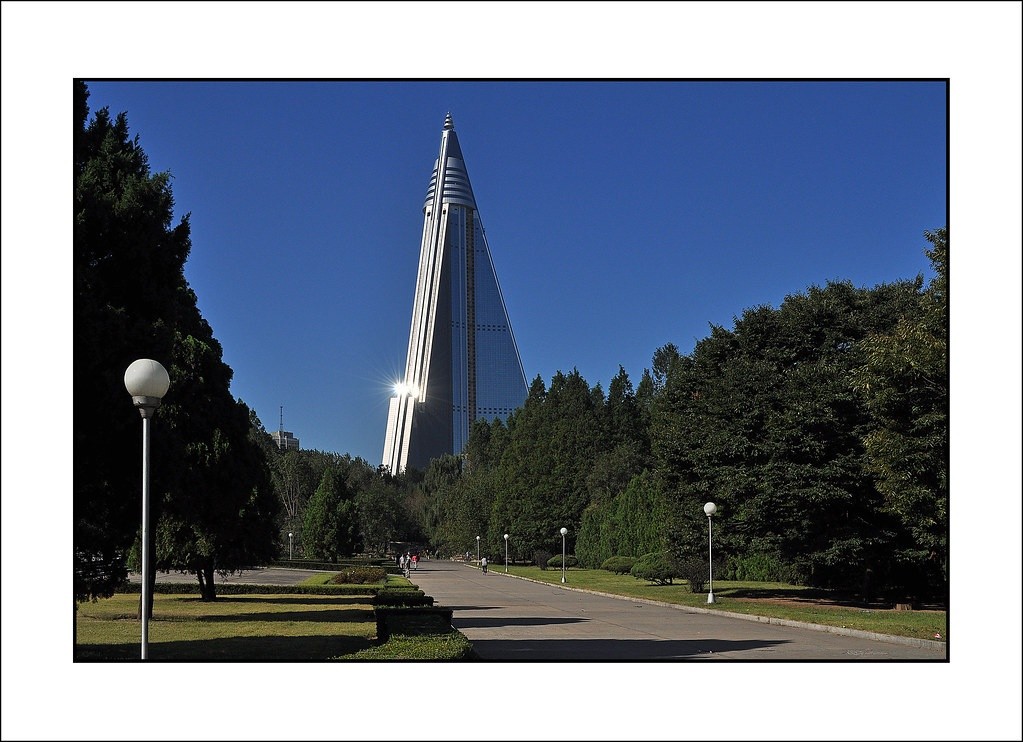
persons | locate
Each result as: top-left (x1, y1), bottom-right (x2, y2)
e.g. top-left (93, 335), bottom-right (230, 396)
top-left (466, 550), bottom-right (470, 558)
top-left (392, 548), bottom-right (444, 572)
top-left (481, 556), bottom-right (489, 573)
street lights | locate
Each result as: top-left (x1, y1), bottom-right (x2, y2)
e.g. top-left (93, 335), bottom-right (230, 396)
top-left (560, 527), bottom-right (568, 584)
top-left (504, 533), bottom-right (509, 574)
top-left (123, 358), bottom-right (172, 661)
top-left (288, 533), bottom-right (293, 560)
top-left (703, 502), bottom-right (717, 604)
top-left (476, 536), bottom-right (480, 567)
